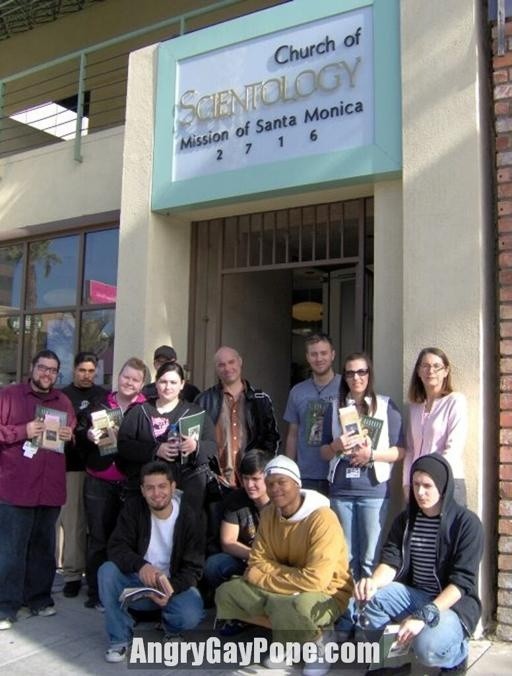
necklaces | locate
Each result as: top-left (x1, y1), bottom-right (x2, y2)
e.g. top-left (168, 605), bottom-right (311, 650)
top-left (311, 369), bottom-right (336, 395)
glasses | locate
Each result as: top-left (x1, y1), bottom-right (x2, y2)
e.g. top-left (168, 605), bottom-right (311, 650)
top-left (79, 368), bottom-right (96, 374)
top-left (345, 369), bottom-right (369, 377)
top-left (422, 362), bottom-right (446, 372)
top-left (38, 364), bottom-right (57, 374)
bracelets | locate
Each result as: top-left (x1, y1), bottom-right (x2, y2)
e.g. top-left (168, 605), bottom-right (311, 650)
top-left (368, 449), bottom-right (375, 463)
top-left (413, 602), bottom-right (440, 628)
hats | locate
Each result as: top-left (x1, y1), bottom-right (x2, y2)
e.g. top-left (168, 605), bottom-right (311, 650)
top-left (411, 458), bottom-right (448, 494)
top-left (265, 454), bottom-right (302, 484)
top-left (154, 345), bottom-right (177, 361)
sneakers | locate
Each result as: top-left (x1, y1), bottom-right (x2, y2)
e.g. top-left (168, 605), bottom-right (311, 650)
top-left (63, 580), bottom-right (81, 598)
top-left (0, 620), bottom-right (12, 630)
top-left (303, 629), bottom-right (338, 676)
top-left (35, 606), bottom-right (55, 616)
top-left (105, 641), bottom-right (133, 663)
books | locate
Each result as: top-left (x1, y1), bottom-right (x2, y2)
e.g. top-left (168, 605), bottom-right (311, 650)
top-left (305, 400), bottom-right (339, 446)
top-left (178, 410), bottom-right (206, 465)
top-left (369, 624), bottom-right (412, 672)
top-left (117, 574), bottom-right (174, 609)
top-left (339, 404), bottom-right (383, 469)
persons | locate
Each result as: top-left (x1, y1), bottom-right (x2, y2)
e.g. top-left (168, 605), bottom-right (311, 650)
top-left (192, 346), bottom-right (282, 460)
top-left (2, 346), bottom-right (200, 630)
top-left (400, 346), bottom-right (470, 509)
top-left (202, 448), bottom-right (272, 634)
top-left (215, 454), bottom-right (355, 675)
top-left (319, 351), bottom-right (407, 581)
top-left (281, 331), bottom-right (344, 496)
top-left (309, 413), bottom-right (324, 441)
top-left (357, 426), bottom-right (372, 449)
top-left (352, 452), bottom-right (483, 676)
top-left (96, 461), bottom-right (207, 663)
